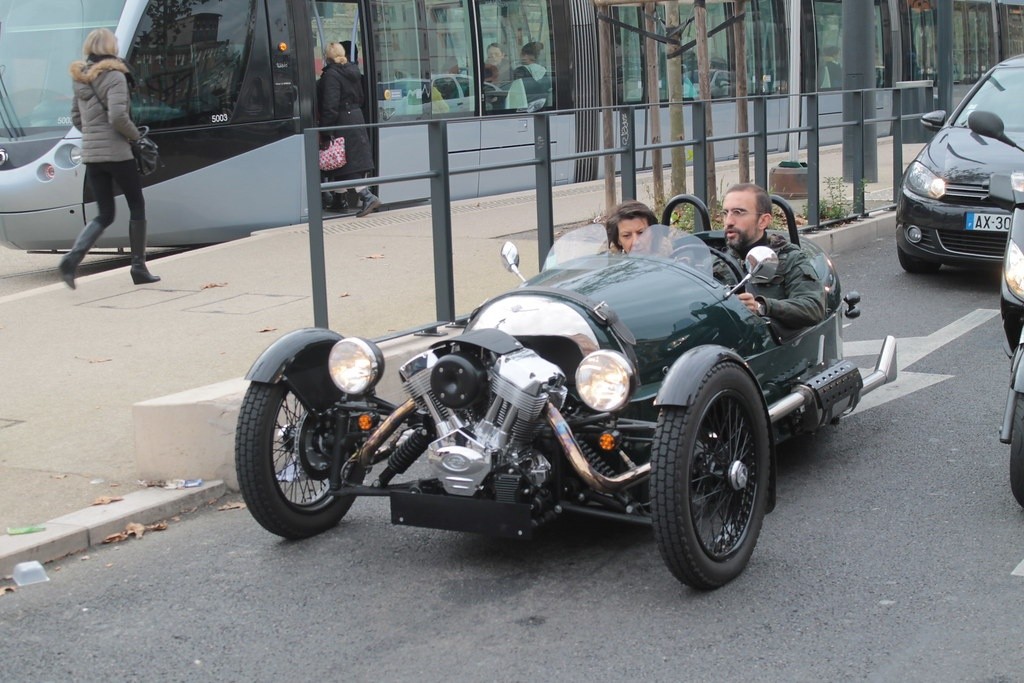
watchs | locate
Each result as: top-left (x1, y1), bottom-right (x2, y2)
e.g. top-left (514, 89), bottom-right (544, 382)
top-left (756, 299), bottom-right (767, 316)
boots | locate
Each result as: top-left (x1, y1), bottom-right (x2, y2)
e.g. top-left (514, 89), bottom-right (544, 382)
top-left (325, 192), bottom-right (349, 214)
top-left (355, 186), bottom-right (381, 218)
top-left (129, 219), bottom-right (160, 285)
top-left (57, 220), bottom-right (104, 290)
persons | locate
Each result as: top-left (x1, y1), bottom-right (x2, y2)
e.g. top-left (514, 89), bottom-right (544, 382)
top-left (316, 38), bottom-right (381, 217)
top-left (59, 26), bottom-right (161, 290)
top-left (681, 37), bottom-right (699, 98)
top-left (598, 200), bottom-right (676, 258)
top-left (702, 182), bottom-right (827, 346)
top-left (432, 41), bottom-right (551, 115)
top-left (823, 45), bottom-right (841, 87)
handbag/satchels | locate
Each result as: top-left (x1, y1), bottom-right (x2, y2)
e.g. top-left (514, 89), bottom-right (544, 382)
top-left (318, 136), bottom-right (347, 171)
top-left (127, 125), bottom-right (165, 177)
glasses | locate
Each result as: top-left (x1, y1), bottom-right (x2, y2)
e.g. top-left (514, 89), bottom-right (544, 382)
top-left (719, 208), bottom-right (764, 220)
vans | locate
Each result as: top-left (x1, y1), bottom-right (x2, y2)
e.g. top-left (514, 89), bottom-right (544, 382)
top-left (896, 53), bottom-right (1024, 273)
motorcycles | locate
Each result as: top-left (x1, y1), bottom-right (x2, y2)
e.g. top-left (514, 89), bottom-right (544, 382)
top-left (989, 172), bottom-right (1024, 506)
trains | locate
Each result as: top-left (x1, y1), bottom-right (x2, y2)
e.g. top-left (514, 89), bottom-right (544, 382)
top-left (0, 0), bottom-right (1024, 255)
